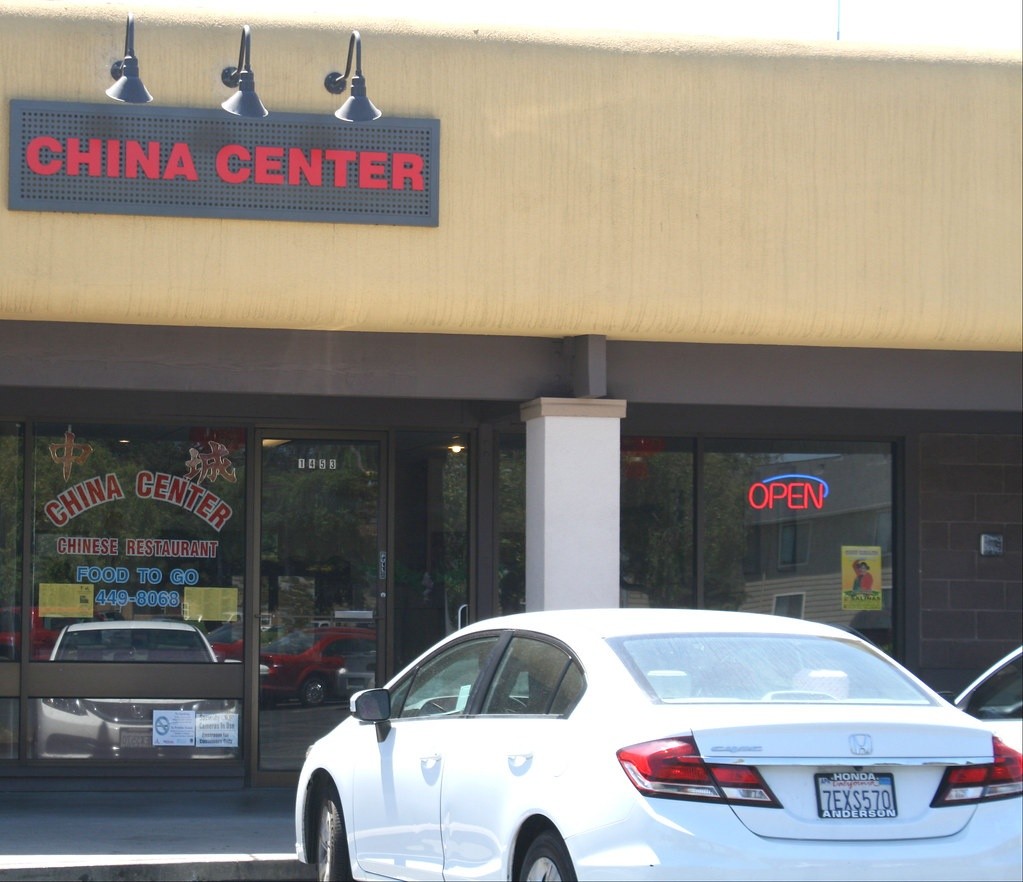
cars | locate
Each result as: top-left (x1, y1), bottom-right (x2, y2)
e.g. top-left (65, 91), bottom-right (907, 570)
top-left (295, 608), bottom-right (1023, 882)
top-left (0, 601), bottom-right (379, 706)
top-left (921, 645), bottom-right (1022, 761)
top-left (31, 619), bottom-right (272, 780)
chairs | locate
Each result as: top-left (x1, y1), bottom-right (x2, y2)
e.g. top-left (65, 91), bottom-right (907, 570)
top-left (793, 668), bottom-right (849, 700)
top-left (644, 671), bottom-right (691, 699)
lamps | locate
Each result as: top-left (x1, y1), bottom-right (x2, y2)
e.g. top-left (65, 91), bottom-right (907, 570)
top-left (324, 32), bottom-right (382, 123)
top-left (221, 25), bottom-right (269, 118)
top-left (448, 429), bottom-right (469, 452)
top-left (106, 13), bottom-right (154, 103)
